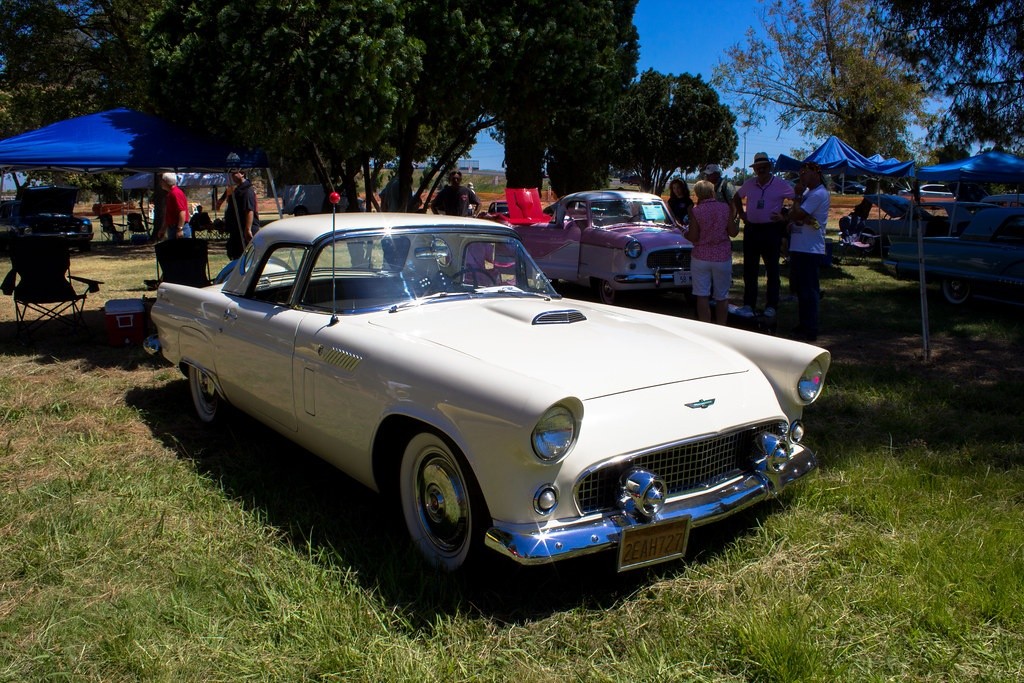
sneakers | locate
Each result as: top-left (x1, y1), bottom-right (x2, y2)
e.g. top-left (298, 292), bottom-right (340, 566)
top-left (735, 304), bottom-right (753, 314)
top-left (764, 307), bottom-right (776, 316)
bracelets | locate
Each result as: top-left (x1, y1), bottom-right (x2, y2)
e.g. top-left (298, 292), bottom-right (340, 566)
top-left (178, 227), bottom-right (183, 231)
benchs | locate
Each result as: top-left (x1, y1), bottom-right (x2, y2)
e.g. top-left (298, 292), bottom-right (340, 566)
top-left (253, 276), bottom-right (412, 308)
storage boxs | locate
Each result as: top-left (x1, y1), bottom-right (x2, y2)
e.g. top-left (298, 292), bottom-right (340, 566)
top-left (105, 299), bottom-right (143, 346)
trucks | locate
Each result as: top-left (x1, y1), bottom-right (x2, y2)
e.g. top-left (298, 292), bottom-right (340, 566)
top-left (281, 184), bottom-right (366, 217)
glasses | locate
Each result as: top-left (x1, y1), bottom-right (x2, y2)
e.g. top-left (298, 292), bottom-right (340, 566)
top-left (799, 170), bottom-right (816, 175)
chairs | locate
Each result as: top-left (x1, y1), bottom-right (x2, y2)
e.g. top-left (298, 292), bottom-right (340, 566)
top-left (834, 215), bottom-right (879, 264)
top-left (0, 236), bottom-right (104, 343)
top-left (127, 213), bottom-right (153, 235)
top-left (448, 242), bottom-right (516, 286)
top-left (154, 237), bottom-right (211, 290)
top-left (99, 213), bottom-right (127, 241)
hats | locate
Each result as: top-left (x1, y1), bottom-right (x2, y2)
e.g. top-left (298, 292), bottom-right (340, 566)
top-left (703, 164), bottom-right (719, 174)
top-left (468, 183), bottom-right (474, 189)
top-left (749, 152), bottom-right (775, 167)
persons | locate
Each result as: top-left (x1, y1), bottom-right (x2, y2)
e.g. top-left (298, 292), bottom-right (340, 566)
top-left (189, 205), bottom-right (212, 239)
top-left (213, 225), bottom-right (294, 285)
top-left (665, 178), bottom-right (695, 224)
top-left (731, 151), bottom-right (796, 317)
top-left (787, 162), bottom-right (830, 334)
top-left (158, 172), bottom-right (193, 239)
top-left (702, 165), bottom-right (737, 219)
top-left (431, 170), bottom-right (481, 218)
top-left (338, 163), bottom-right (425, 270)
top-left (675, 180), bottom-right (740, 325)
top-left (217, 171), bottom-right (260, 260)
top-left (467, 182), bottom-right (475, 216)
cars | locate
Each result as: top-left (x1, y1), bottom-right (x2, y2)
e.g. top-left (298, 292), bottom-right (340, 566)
top-left (149, 211), bottom-right (832, 590)
top-left (0, 183), bottom-right (95, 255)
top-left (883, 193), bottom-right (1023, 313)
top-left (464, 189), bottom-right (695, 306)
top-left (898, 184), bottom-right (955, 198)
top-left (834, 181), bottom-right (867, 195)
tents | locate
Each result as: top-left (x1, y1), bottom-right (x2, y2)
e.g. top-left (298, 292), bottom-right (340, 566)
top-left (120, 170), bottom-right (236, 230)
top-left (912, 151), bottom-right (1023, 237)
top-left (776, 134), bottom-right (916, 264)
top-left (0, 107), bottom-right (299, 270)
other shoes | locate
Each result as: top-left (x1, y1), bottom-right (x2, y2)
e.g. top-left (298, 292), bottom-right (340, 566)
top-left (144, 279), bottom-right (161, 288)
top-left (792, 328), bottom-right (816, 340)
